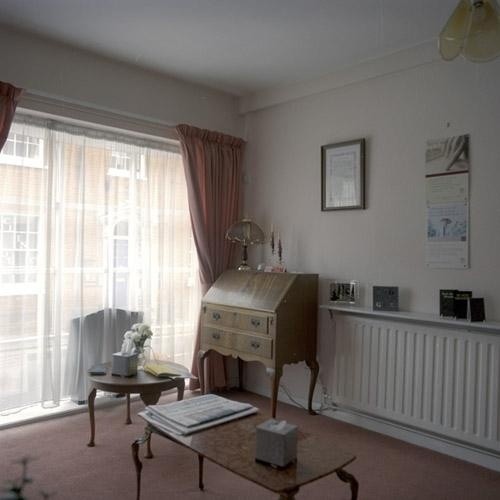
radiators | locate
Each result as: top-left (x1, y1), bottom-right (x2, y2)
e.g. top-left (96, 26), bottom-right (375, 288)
top-left (319, 301), bottom-right (500, 453)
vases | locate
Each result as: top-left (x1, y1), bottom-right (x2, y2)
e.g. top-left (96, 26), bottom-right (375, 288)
top-left (133, 346), bottom-right (146, 371)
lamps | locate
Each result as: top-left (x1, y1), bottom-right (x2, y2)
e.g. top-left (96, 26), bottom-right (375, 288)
top-left (437, 0), bottom-right (500, 64)
top-left (224, 220), bottom-right (265, 270)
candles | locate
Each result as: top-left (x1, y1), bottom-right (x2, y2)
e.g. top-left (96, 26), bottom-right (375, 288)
top-left (278, 231), bottom-right (282, 262)
top-left (271, 222), bottom-right (275, 257)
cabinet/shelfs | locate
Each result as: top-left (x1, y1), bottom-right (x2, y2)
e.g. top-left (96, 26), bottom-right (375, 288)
top-left (195, 270), bottom-right (319, 418)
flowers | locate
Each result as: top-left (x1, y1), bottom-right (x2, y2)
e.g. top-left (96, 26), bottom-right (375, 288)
top-left (123, 322), bottom-right (153, 351)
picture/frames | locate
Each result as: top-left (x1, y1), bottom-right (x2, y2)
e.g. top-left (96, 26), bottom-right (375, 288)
top-left (319, 138), bottom-right (366, 212)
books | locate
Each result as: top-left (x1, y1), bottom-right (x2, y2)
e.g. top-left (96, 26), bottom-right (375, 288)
top-left (373, 285), bottom-right (400, 312)
top-left (453, 291), bottom-right (472, 319)
top-left (470, 297), bottom-right (485, 322)
top-left (439, 289), bottom-right (459, 318)
top-left (143, 363), bottom-right (199, 380)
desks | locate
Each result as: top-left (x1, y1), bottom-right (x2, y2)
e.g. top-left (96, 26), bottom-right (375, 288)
top-left (88, 356), bottom-right (183, 446)
